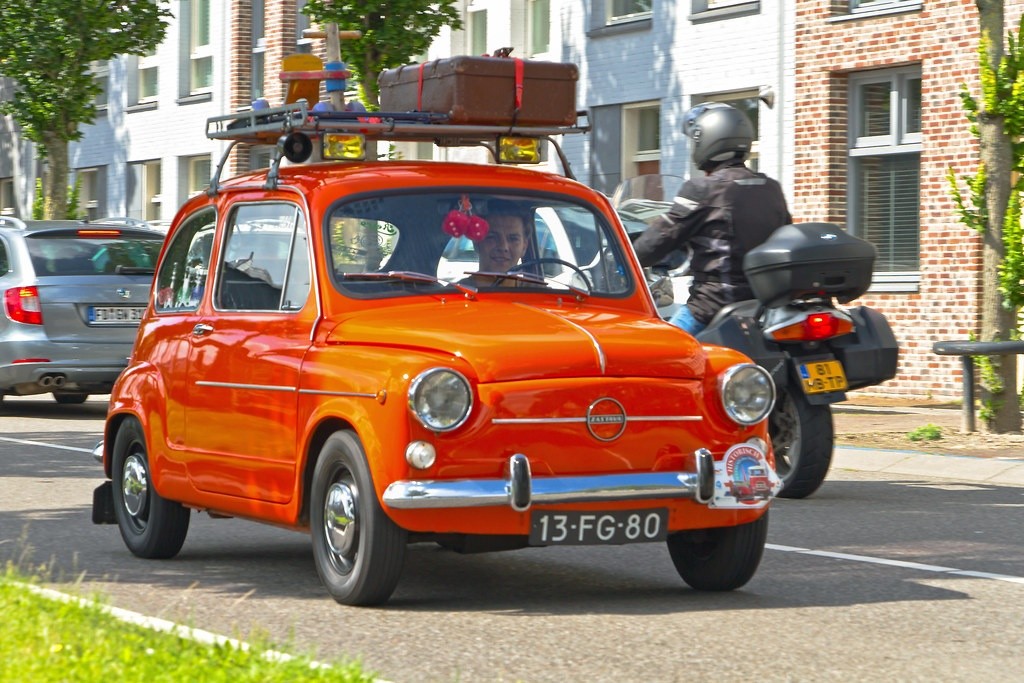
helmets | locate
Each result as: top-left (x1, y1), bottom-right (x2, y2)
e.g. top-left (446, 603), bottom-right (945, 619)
top-left (681, 102), bottom-right (755, 171)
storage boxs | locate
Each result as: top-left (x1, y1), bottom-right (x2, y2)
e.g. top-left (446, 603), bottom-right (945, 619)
top-left (376, 56), bottom-right (579, 126)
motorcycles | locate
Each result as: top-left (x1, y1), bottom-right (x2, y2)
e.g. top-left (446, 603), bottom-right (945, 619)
top-left (589, 171), bottom-right (901, 501)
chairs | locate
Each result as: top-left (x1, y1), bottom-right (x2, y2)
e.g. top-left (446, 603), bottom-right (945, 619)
top-left (54, 248), bottom-right (96, 273)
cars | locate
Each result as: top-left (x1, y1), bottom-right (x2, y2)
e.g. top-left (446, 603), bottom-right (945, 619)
top-left (84, 106), bottom-right (786, 610)
top-left (377, 201), bottom-right (695, 306)
top-left (0, 211), bottom-right (310, 403)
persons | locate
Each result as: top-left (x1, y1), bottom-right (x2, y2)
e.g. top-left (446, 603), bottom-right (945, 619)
top-left (614, 101), bottom-right (792, 336)
top-left (446, 201), bottom-right (547, 291)
